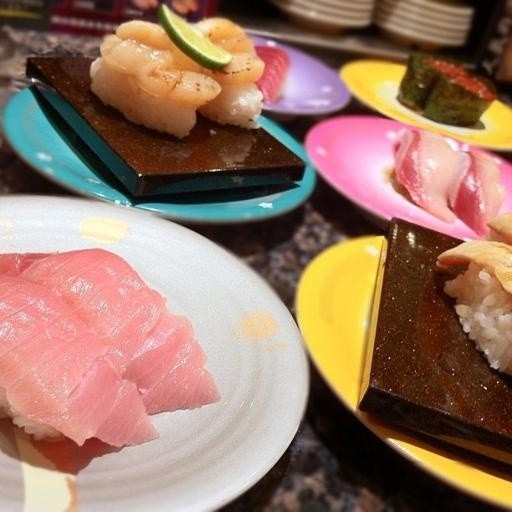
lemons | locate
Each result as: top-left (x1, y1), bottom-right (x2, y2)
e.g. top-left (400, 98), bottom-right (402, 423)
top-left (161, 3), bottom-right (234, 70)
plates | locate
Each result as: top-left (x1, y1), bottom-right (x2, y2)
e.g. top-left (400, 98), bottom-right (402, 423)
top-left (296, 231), bottom-right (510, 508)
top-left (280, 2), bottom-right (473, 53)
top-left (4, 86), bottom-right (318, 221)
top-left (0, 203), bottom-right (309, 506)
top-left (241, 31), bottom-right (354, 118)
top-left (303, 116), bottom-right (511, 245)
top-left (338, 58), bottom-right (512, 153)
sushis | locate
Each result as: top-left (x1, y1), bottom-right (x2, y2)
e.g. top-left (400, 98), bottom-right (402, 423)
top-left (0, 249), bottom-right (220, 449)
top-left (432, 241), bottom-right (512, 375)
top-left (390, 131), bottom-right (504, 236)
top-left (255, 45), bottom-right (290, 104)
top-left (399, 51), bottom-right (498, 125)
